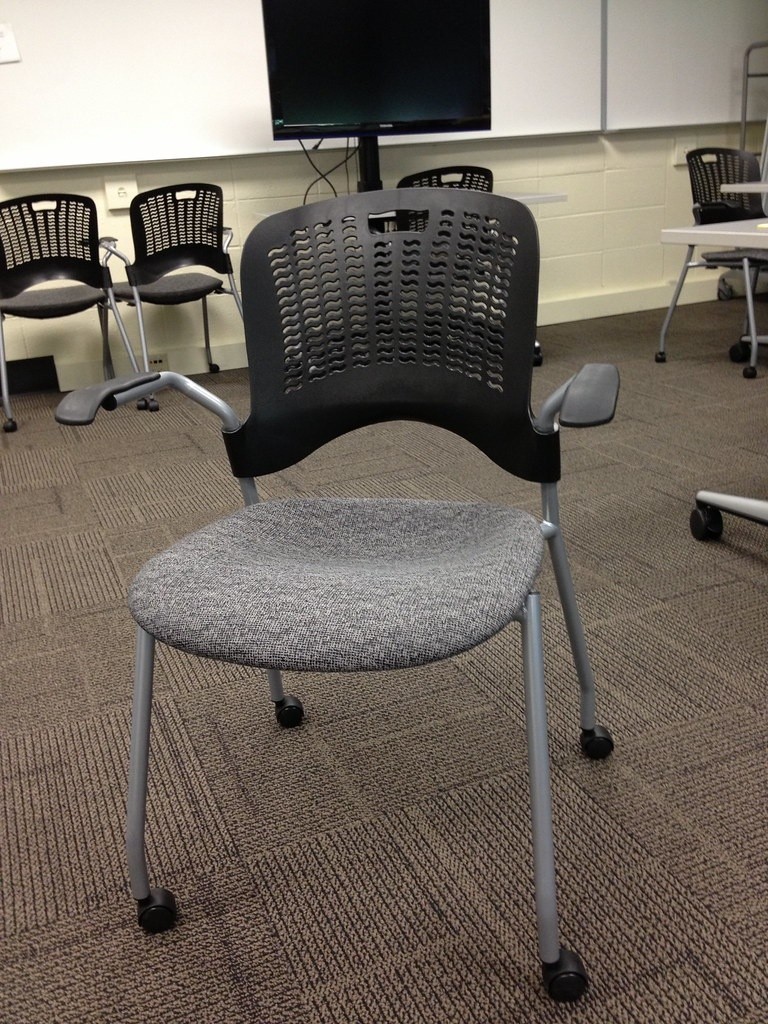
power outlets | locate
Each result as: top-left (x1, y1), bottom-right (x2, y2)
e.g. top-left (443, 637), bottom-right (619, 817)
top-left (130, 352), bottom-right (168, 381)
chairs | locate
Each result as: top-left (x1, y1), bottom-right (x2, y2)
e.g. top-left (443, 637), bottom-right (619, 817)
top-left (99, 183), bottom-right (244, 412)
top-left (654, 146), bottom-right (768, 379)
top-left (127, 189), bottom-right (613, 1002)
top-left (0, 195), bottom-right (146, 433)
top-left (393, 166), bottom-right (543, 366)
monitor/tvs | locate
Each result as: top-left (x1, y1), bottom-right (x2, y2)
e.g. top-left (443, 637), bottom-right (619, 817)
top-left (261, 0), bottom-right (491, 141)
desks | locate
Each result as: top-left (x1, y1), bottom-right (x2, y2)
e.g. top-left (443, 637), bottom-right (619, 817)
top-left (662, 218), bottom-right (768, 363)
